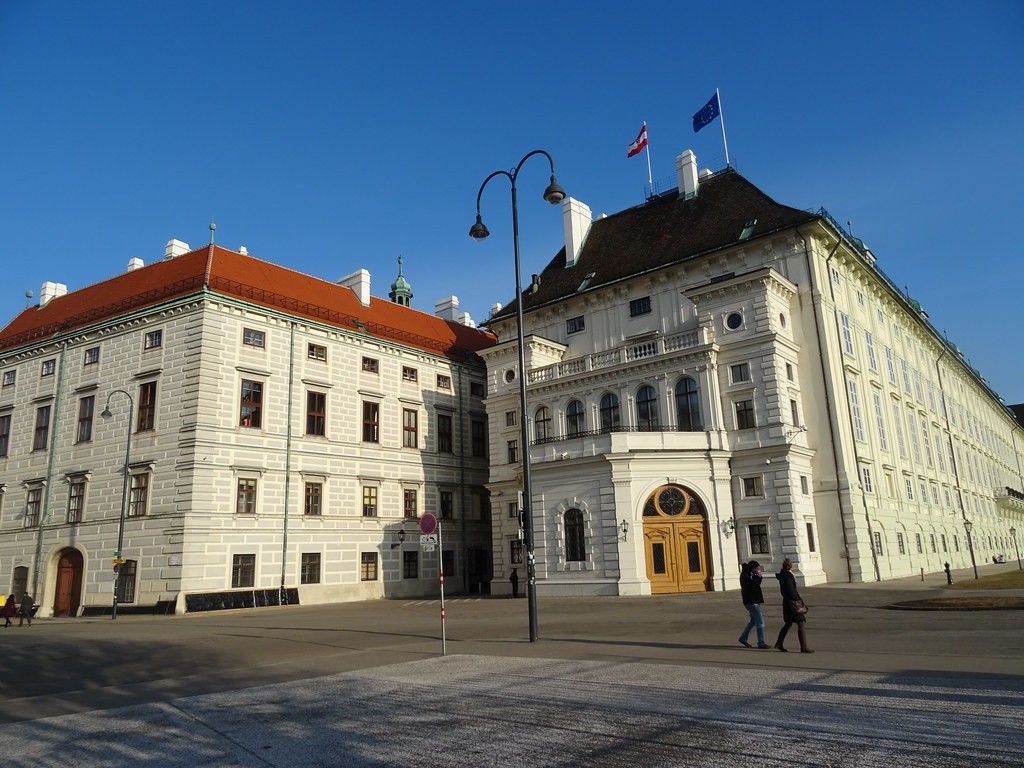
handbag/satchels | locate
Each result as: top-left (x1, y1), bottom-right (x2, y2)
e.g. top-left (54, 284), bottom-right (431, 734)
top-left (790, 600), bottom-right (808, 615)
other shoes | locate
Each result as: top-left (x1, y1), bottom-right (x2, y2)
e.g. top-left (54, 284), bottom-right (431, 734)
top-left (774, 642), bottom-right (789, 652)
top-left (758, 642), bottom-right (771, 649)
top-left (801, 648), bottom-right (815, 653)
top-left (739, 638), bottom-right (752, 647)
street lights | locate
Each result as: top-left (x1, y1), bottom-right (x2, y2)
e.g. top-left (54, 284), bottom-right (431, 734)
top-left (468, 150), bottom-right (566, 644)
top-left (100, 388), bottom-right (135, 621)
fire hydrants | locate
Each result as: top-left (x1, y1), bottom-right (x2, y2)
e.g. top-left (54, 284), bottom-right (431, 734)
top-left (943, 560), bottom-right (955, 584)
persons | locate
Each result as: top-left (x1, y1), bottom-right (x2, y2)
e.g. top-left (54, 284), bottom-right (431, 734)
top-left (17, 592), bottom-right (32, 627)
top-left (510, 568), bottom-right (518, 597)
top-left (739, 561), bottom-right (771, 649)
top-left (2, 594), bottom-right (15, 628)
top-left (774, 559), bottom-right (815, 653)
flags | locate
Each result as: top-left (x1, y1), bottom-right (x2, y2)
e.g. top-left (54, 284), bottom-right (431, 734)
top-left (693, 93), bottom-right (721, 132)
top-left (627, 125), bottom-right (649, 157)
